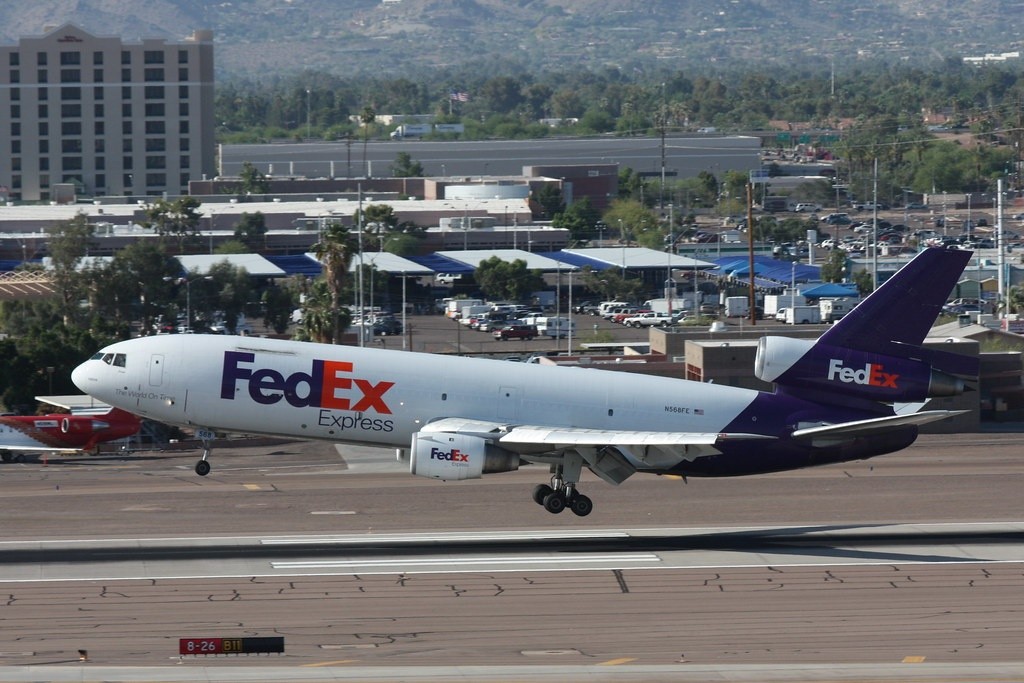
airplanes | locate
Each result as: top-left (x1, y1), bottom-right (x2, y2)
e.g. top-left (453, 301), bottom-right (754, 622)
top-left (71, 245), bottom-right (975, 517)
top-left (0, 394), bottom-right (143, 459)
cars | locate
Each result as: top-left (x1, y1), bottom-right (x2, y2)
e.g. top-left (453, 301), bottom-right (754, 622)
top-left (350, 307), bottom-right (403, 336)
top-left (571, 301), bottom-right (672, 329)
top-left (130, 313), bottom-right (250, 336)
top-left (493, 325), bottom-right (538, 341)
top-left (690, 203), bottom-right (1023, 258)
top-left (673, 302), bottom-right (715, 326)
top-left (465, 303), bottom-right (544, 329)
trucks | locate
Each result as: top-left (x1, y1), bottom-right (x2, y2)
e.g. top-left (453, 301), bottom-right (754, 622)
top-left (447, 300), bottom-right (490, 320)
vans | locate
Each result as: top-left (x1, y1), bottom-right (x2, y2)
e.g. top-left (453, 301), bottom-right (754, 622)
top-left (536, 318), bottom-right (575, 339)
